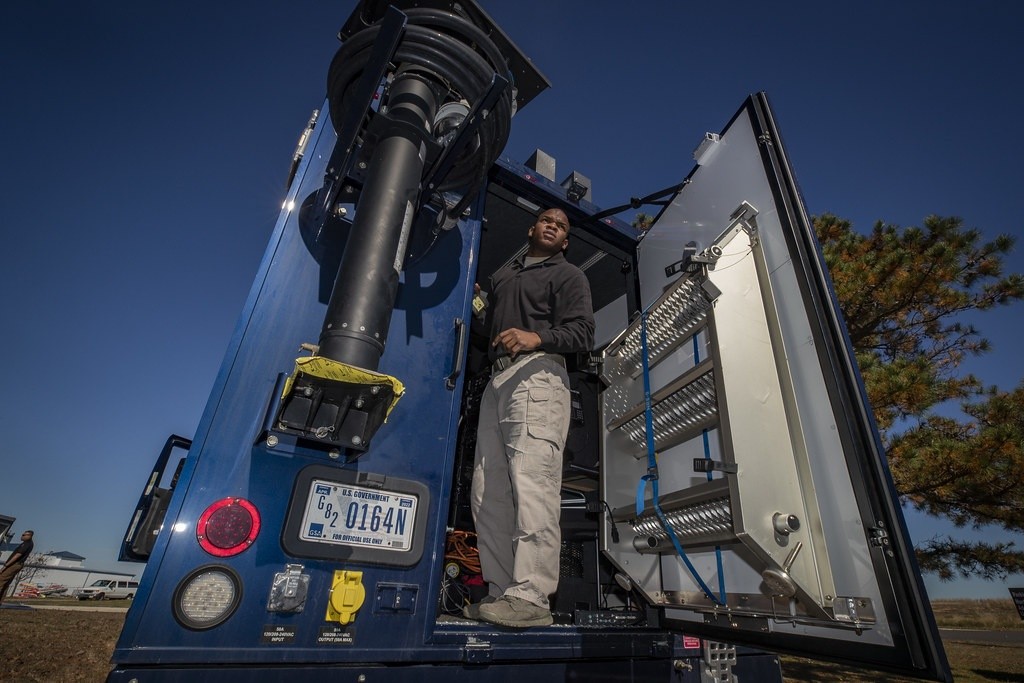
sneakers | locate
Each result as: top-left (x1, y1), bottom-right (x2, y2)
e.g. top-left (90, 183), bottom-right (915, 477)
top-left (479, 595), bottom-right (553, 628)
top-left (463, 594), bottom-right (496, 621)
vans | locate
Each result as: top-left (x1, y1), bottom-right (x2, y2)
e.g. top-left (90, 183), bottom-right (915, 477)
top-left (77, 579), bottom-right (138, 600)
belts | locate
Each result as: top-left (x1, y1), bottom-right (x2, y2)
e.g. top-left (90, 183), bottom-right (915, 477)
top-left (491, 353), bottom-right (565, 376)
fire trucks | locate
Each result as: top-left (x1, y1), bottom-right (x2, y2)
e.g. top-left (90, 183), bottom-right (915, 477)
top-left (106, 1), bottom-right (958, 683)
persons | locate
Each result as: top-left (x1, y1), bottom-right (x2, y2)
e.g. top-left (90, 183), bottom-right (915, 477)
top-left (464, 208), bottom-right (595, 627)
top-left (0, 529), bottom-right (35, 600)
